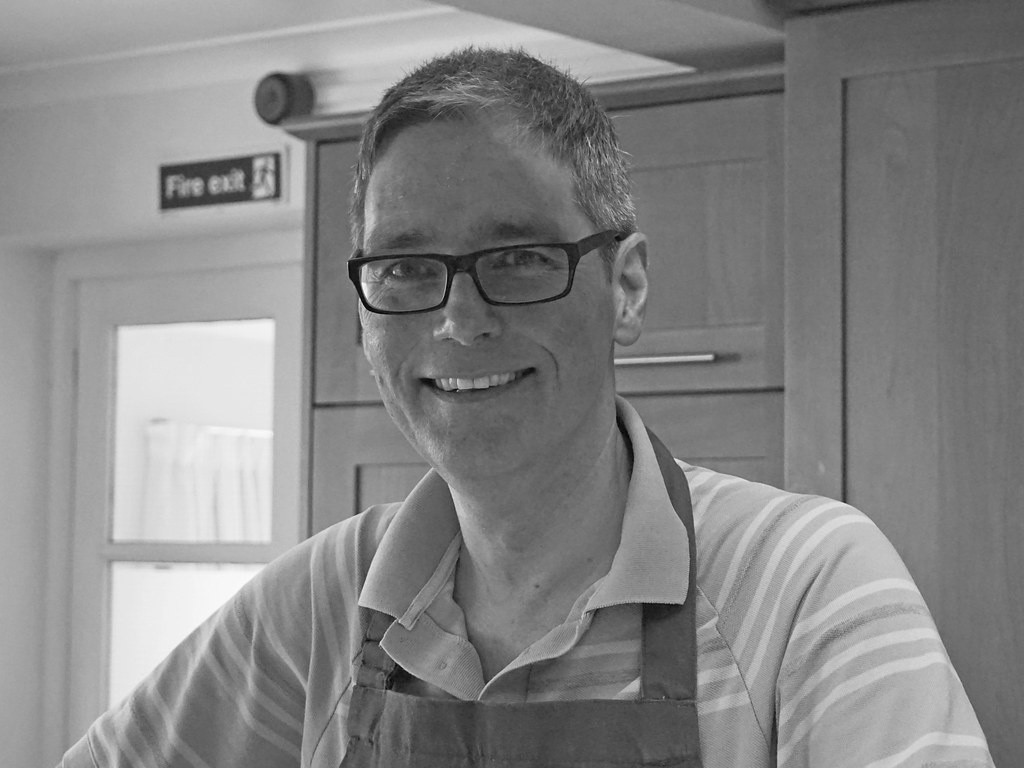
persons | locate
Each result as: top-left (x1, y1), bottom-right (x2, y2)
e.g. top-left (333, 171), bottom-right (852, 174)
top-left (58, 44), bottom-right (996, 766)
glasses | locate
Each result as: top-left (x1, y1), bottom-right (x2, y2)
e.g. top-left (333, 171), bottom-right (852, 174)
top-left (348, 230), bottom-right (628, 315)
